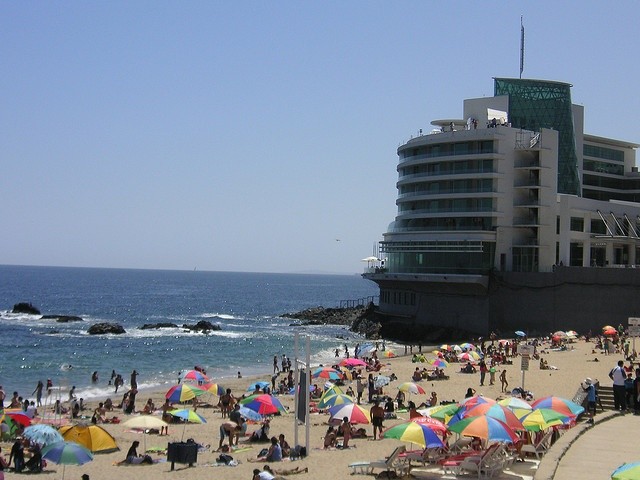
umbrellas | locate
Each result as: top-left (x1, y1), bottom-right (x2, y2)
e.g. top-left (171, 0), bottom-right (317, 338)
top-left (323, 394), bottom-right (356, 405)
top-left (603, 325), bottom-right (617, 339)
top-left (499, 340), bottom-right (511, 346)
top-left (22, 424), bottom-right (64, 450)
top-left (463, 402), bottom-right (531, 431)
top-left (317, 384), bottom-right (345, 408)
top-left (431, 343), bottom-right (485, 368)
top-left (239, 394), bottom-right (289, 427)
top-left (459, 396), bottom-right (496, 408)
top-left (199, 382), bottom-right (226, 402)
top-left (382, 349), bottom-right (398, 358)
top-left (166, 409), bottom-right (208, 441)
top-left (338, 357), bottom-right (369, 384)
top-left (515, 330), bottom-right (526, 337)
top-left (41, 440), bottom-right (93, 480)
top-left (178, 370), bottom-right (210, 384)
top-left (397, 382), bottom-right (426, 402)
top-left (328, 403), bottom-right (373, 424)
top-left (498, 398), bottom-right (533, 411)
top-left (553, 330), bottom-right (579, 349)
top-left (165, 384), bottom-right (207, 409)
top-left (356, 343), bottom-right (374, 356)
top-left (514, 408), bottom-right (571, 432)
top-left (416, 404), bottom-right (462, 419)
top-left (2, 407), bottom-right (31, 436)
top-left (533, 395), bottom-right (584, 424)
top-left (409, 416), bottom-right (446, 431)
top-left (312, 367), bottom-right (344, 385)
top-left (380, 421), bottom-right (446, 464)
top-left (247, 381), bottom-right (271, 393)
top-left (123, 415), bottom-right (167, 455)
top-left (448, 415), bottom-right (520, 456)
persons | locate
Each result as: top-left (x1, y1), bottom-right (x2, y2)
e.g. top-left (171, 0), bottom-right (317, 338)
top-left (372, 401), bottom-right (387, 440)
top-left (335, 322), bottom-right (395, 396)
top-left (252, 419), bottom-right (310, 480)
top-left (579, 377), bottom-right (599, 425)
top-left (607, 360), bottom-right (639, 416)
top-left (218, 391), bottom-right (248, 470)
top-left (586, 322), bottom-right (640, 360)
top-left (526, 328), bottom-right (559, 370)
top-left (398, 343), bottom-right (448, 382)
top-left (0, 368), bottom-right (155, 424)
top-left (482, 337), bottom-right (508, 391)
top-left (324, 416), bottom-right (371, 455)
top-left (177, 353), bottom-right (311, 389)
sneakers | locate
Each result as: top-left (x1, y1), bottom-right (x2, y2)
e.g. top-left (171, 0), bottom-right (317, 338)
top-left (587, 419), bottom-right (593, 423)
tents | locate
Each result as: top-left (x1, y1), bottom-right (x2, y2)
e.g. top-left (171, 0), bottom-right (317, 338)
top-left (58, 423), bottom-right (121, 455)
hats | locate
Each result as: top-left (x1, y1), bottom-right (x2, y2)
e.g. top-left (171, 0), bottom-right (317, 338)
top-left (584, 378), bottom-right (592, 384)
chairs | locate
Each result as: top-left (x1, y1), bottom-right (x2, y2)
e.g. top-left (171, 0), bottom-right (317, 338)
top-left (366, 445), bottom-right (405, 475)
top-left (349, 461), bottom-right (370, 474)
top-left (520, 430), bottom-right (553, 459)
top-left (408, 435), bottom-right (473, 465)
top-left (443, 442), bottom-right (519, 480)
